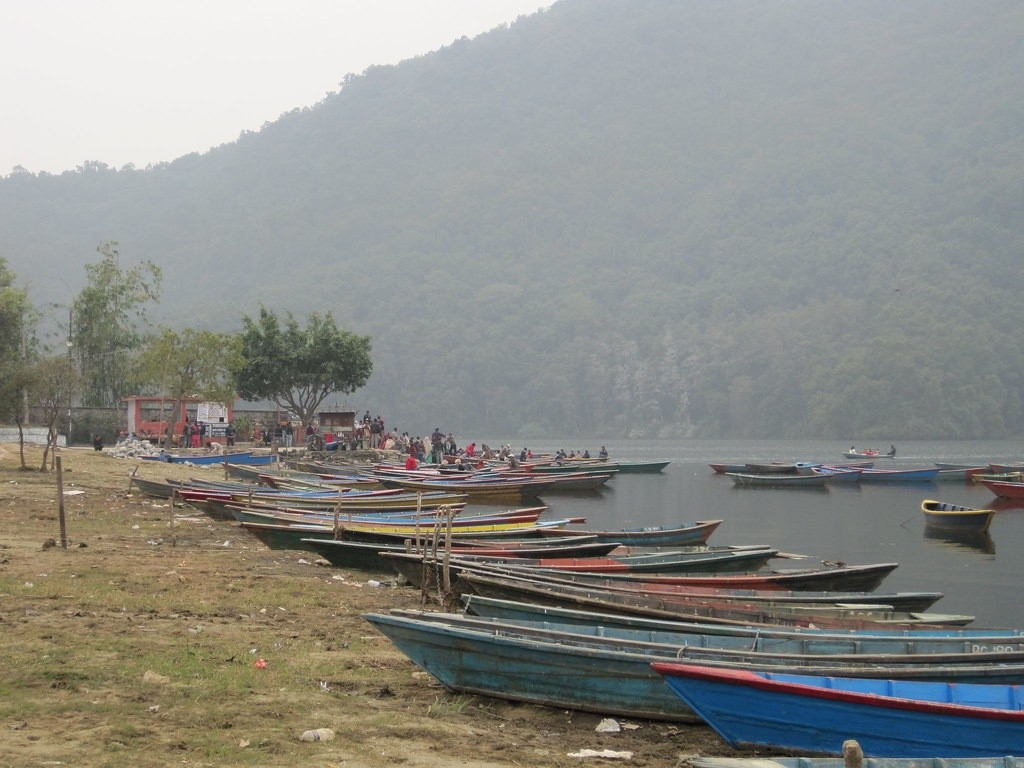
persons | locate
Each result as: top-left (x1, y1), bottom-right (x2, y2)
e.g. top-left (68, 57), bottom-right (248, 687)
top-left (862, 447), bottom-right (881, 456)
top-left (92, 433), bottom-right (104, 452)
top-left (850, 445), bottom-right (858, 455)
top-left (114, 406), bottom-right (613, 471)
top-left (887, 443), bottom-right (896, 455)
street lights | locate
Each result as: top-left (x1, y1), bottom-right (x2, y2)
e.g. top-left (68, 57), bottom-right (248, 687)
top-left (67, 334), bottom-right (75, 420)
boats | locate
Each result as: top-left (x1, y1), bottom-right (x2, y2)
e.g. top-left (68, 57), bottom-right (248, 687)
top-left (361, 612), bottom-right (1023, 725)
top-left (650, 656), bottom-right (1023, 758)
top-left (988, 463), bottom-right (1024, 474)
top-left (922, 499), bottom-right (996, 535)
top-left (387, 609), bottom-right (1023, 664)
top-left (934, 461), bottom-right (1006, 473)
top-left (681, 755), bottom-right (1024, 767)
top-left (972, 471), bottom-right (1024, 484)
top-left (981, 479), bottom-right (1024, 499)
top-left (936, 466), bottom-right (987, 479)
top-left (130, 441), bottom-right (975, 624)
